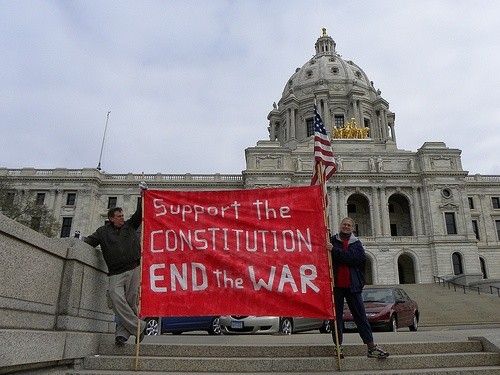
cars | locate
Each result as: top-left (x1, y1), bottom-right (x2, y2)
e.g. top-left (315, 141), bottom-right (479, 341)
top-left (343, 286), bottom-right (421, 333)
top-left (220, 315), bottom-right (331, 335)
top-left (140, 315), bottom-right (219, 336)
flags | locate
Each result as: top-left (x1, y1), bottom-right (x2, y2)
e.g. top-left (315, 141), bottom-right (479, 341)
top-left (309, 104), bottom-right (337, 187)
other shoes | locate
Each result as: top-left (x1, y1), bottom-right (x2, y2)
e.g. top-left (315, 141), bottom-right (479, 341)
top-left (135, 322), bottom-right (148, 344)
top-left (115, 336), bottom-right (125, 345)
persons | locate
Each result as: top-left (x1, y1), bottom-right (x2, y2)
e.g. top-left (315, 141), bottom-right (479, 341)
top-left (72, 181), bottom-right (148, 346)
top-left (368, 156), bottom-right (385, 172)
top-left (327, 217), bottom-right (389, 359)
top-left (295, 154), bottom-right (302, 172)
top-left (336, 155), bottom-right (343, 172)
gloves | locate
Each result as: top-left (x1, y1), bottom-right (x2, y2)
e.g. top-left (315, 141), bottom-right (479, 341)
top-left (138, 181), bottom-right (148, 189)
top-left (72, 231), bottom-right (83, 241)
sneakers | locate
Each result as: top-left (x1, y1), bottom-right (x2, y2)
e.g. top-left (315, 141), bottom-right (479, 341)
top-left (334, 345), bottom-right (345, 358)
top-left (368, 344), bottom-right (390, 358)
